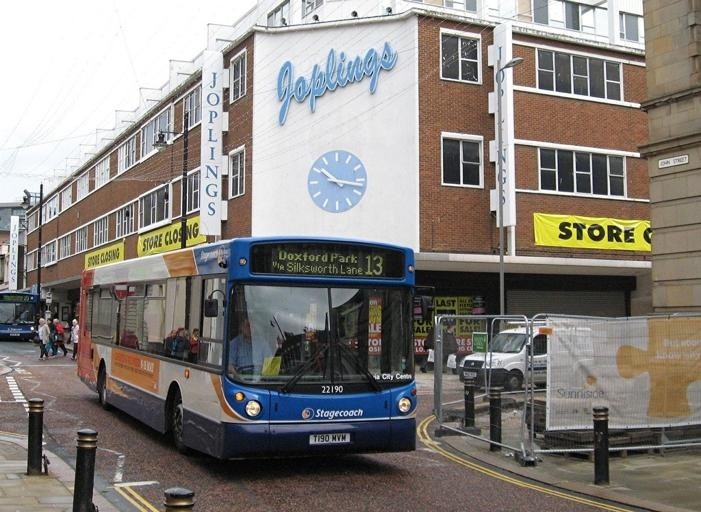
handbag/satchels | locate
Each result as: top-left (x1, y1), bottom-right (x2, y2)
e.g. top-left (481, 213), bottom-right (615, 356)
top-left (34, 335), bottom-right (40, 344)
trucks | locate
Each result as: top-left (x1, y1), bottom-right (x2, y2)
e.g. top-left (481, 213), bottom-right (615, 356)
top-left (459, 326), bottom-right (594, 391)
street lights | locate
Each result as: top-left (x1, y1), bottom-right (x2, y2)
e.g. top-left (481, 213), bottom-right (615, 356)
top-left (495, 56), bottom-right (526, 327)
top-left (20, 184), bottom-right (44, 296)
top-left (151, 110), bottom-right (188, 248)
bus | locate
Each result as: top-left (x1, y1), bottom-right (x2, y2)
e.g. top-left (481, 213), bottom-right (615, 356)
top-left (76, 234), bottom-right (436, 463)
top-left (0, 292), bottom-right (39, 342)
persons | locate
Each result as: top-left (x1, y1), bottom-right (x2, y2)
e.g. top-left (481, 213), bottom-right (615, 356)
top-left (419, 328), bottom-right (434, 373)
top-left (444, 322), bottom-right (459, 374)
top-left (228, 317), bottom-right (273, 376)
top-left (37, 316), bottom-right (80, 361)
top-left (165, 326), bottom-right (200, 364)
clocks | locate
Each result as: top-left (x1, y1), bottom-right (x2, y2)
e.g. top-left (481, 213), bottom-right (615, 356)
top-left (307, 150), bottom-right (367, 213)
